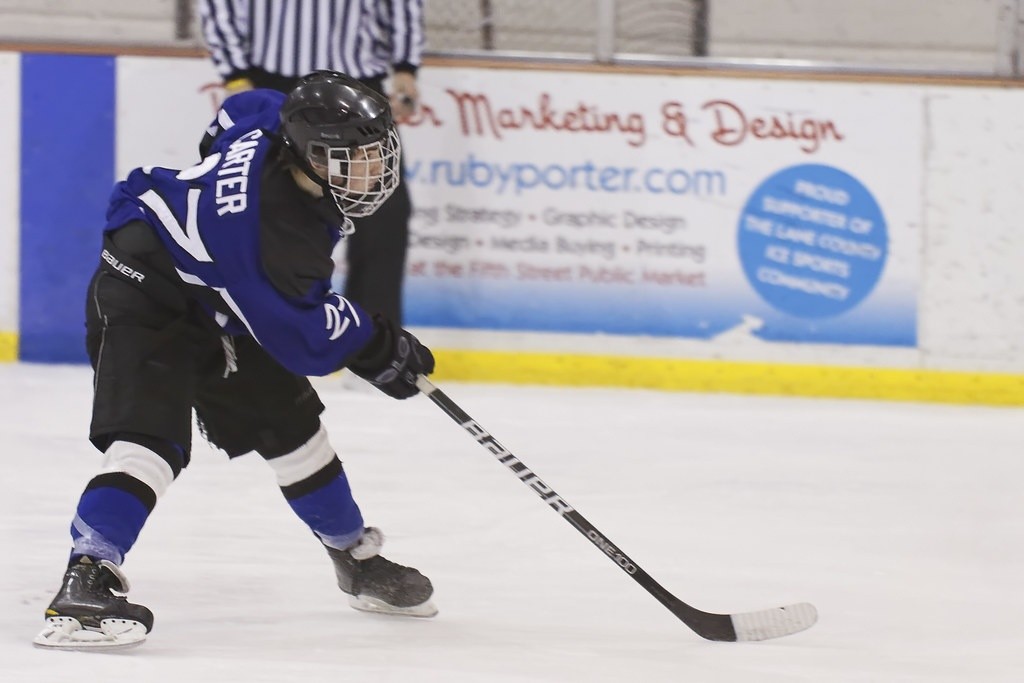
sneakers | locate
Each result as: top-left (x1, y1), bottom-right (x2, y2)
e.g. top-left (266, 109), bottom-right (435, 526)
top-left (326, 526), bottom-right (434, 608)
top-left (45, 552), bottom-right (154, 634)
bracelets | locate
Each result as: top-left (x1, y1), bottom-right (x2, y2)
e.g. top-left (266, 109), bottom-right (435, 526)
top-left (224, 79), bottom-right (248, 92)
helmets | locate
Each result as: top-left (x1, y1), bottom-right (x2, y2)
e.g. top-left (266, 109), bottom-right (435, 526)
top-left (279, 69), bottom-right (393, 170)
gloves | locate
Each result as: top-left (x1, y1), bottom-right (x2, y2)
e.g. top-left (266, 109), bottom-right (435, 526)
top-left (345, 313), bottom-right (435, 400)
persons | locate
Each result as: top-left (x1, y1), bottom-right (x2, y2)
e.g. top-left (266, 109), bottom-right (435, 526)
top-left (201, 0), bottom-right (424, 328)
top-left (31, 69), bottom-right (438, 652)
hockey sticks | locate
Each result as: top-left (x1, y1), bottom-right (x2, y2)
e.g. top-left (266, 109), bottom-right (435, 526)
top-left (415, 373), bottom-right (819, 643)
